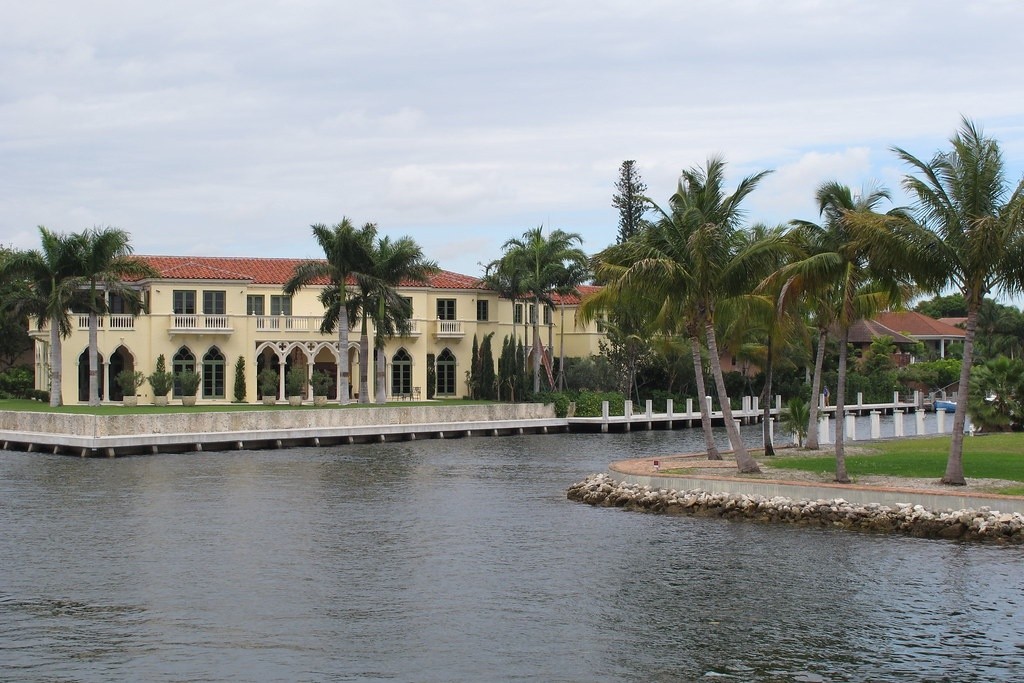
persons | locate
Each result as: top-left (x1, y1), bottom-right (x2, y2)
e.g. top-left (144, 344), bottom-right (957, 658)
top-left (823, 385), bottom-right (830, 406)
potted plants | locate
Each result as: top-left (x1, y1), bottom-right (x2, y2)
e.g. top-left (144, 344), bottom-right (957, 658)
top-left (114, 370), bottom-right (145, 407)
top-left (308, 370), bottom-right (333, 406)
top-left (257, 365), bottom-right (279, 405)
top-left (178, 369), bottom-right (201, 407)
top-left (286, 365), bottom-right (305, 407)
top-left (147, 374), bottom-right (174, 407)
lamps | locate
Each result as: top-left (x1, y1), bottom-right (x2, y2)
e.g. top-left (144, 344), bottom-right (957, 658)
top-left (240, 288), bottom-right (244, 294)
top-left (156, 288), bottom-right (160, 293)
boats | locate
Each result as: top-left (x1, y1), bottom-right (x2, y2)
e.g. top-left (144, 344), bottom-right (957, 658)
top-left (923, 394), bottom-right (958, 413)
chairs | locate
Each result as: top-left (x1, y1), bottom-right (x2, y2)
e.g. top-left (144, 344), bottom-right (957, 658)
top-left (410, 387), bottom-right (423, 401)
top-left (392, 386), bottom-right (406, 401)
